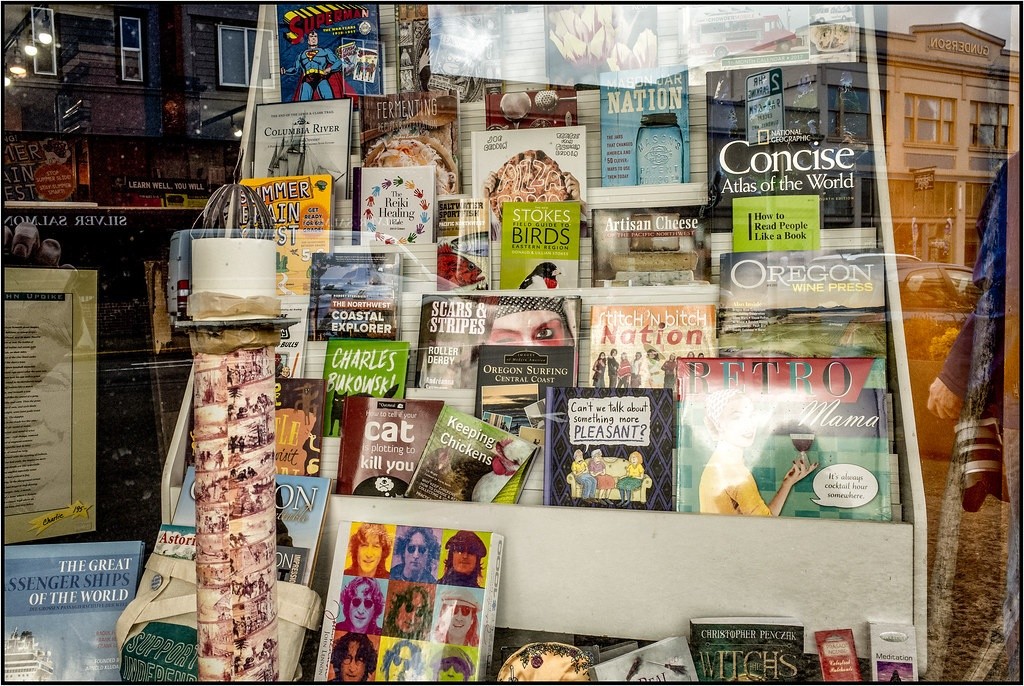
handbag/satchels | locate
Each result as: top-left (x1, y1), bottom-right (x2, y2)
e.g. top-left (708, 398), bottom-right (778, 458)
top-left (116, 553), bottom-right (322, 681)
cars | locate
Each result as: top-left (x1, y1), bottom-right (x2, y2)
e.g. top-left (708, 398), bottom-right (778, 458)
top-left (807, 252), bottom-right (981, 314)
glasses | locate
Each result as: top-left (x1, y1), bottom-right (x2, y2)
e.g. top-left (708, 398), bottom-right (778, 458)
top-left (343, 655), bottom-right (364, 666)
top-left (453, 544), bottom-right (478, 556)
top-left (405, 603), bottom-right (424, 617)
top-left (408, 546), bottom-right (426, 554)
top-left (393, 657), bottom-right (411, 669)
top-left (450, 607), bottom-right (470, 616)
top-left (443, 663), bottom-right (461, 673)
top-left (352, 597), bottom-right (372, 608)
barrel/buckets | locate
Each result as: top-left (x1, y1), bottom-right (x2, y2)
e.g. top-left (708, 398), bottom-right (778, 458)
top-left (191, 238), bottom-right (277, 321)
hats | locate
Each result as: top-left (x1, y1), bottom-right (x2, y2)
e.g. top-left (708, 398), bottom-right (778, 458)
top-left (429, 646), bottom-right (476, 675)
top-left (445, 531), bottom-right (486, 557)
top-left (441, 589), bottom-right (482, 613)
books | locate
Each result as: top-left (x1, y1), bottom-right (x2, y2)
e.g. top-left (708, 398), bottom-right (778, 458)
top-left (119, 2), bottom-right (918, 515)
top-left (689, 617), bottom-right (806, 682)
top-left (588, 636), bottom-right (699, 682)
top-left (3, 467), bottom-right (332, 682)
top-left (314, 520), bottom-right (503, 682)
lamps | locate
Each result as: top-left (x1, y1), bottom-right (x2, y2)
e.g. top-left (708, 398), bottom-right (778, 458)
top-left (5, 8), bottom-right (52, 87)
top-left (230, 116), bottom-right (242, 137)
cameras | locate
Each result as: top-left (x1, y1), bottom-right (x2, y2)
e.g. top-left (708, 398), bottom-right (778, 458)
top-left (954, 402), bottom-right (1004, 513)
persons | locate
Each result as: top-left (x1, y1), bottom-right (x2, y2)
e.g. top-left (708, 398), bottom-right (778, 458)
top-left (3, 222), bottom-right (78, 271)
top-left (703, 390), bottom-right (817, 517)
top-left (326, 523), bottom-right (487, 682)
top-left (928, 149), bottom-right (1019, 681)
